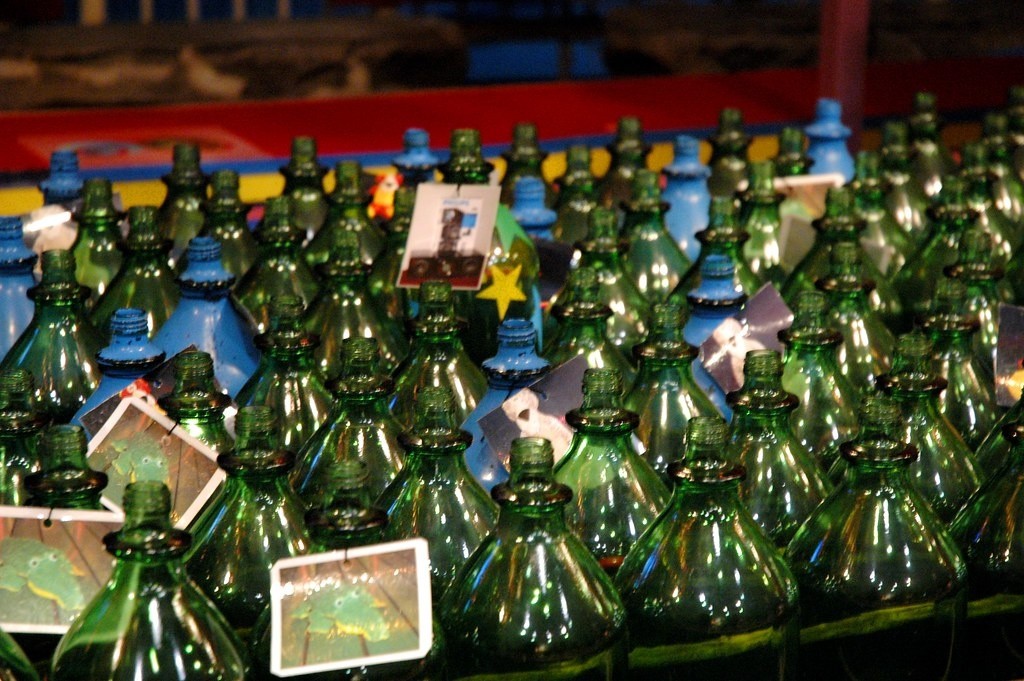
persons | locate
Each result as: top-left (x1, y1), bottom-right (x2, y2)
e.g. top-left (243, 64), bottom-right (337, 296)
top-left (179, 40), bottom-right (415, 98)
top-left (30, 206), bottom-right (77, 275)
top-left (501, 388), bottom-right (571, 464)
top-left (714, 316), bottom-right (766, 389)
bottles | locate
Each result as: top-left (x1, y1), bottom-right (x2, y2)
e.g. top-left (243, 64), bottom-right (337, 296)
top-left (0, 95), bottom-right (1024, 681)
